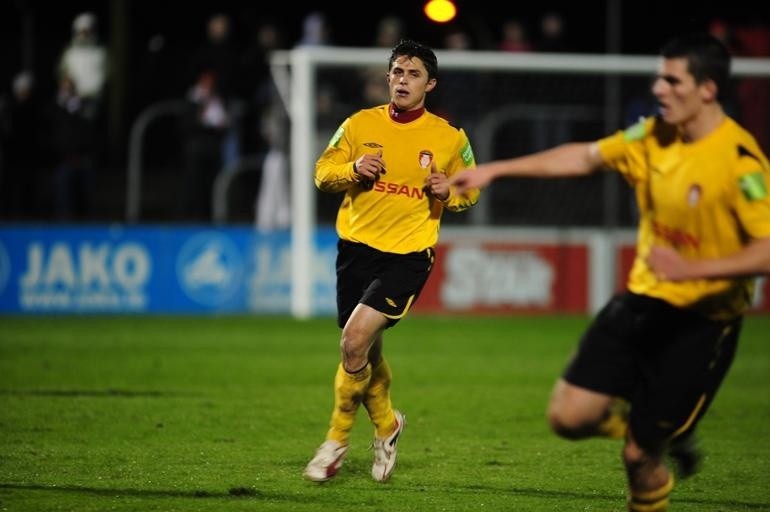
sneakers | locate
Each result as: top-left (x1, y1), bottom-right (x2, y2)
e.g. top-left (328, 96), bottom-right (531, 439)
top-left (671, 423), bottom-right (705, 481)
top-left (372, 408), bottom-right (404, 482)
top-left (303, 440), bottom-right (349, 483)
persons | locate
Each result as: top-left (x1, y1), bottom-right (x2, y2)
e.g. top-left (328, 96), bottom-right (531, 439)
top-left (302, 41), bottom-right (480, 484)
top-left (450, 38), bottom-right (769, 511)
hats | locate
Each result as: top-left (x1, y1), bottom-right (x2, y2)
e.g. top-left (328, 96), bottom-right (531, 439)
top-left (72, 14), bottom-right (99, 33)
